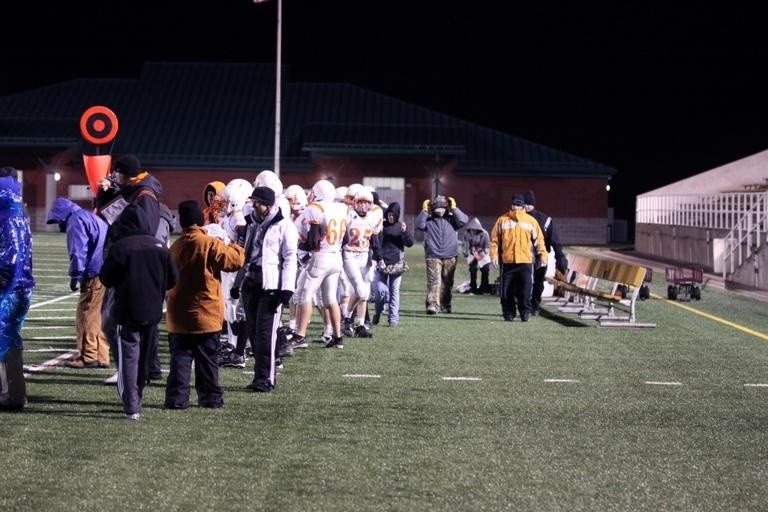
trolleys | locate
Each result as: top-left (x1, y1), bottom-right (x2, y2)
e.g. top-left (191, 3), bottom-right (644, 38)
top-left (666, 263), bottom-right (703, 301)
top-left (615, 267), bottom-right (653, 299)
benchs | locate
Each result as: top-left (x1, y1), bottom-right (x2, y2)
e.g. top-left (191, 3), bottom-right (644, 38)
top-left (654, 171), bottom-right (768, 231)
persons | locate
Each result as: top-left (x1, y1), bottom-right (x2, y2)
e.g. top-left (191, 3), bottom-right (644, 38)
top-left (47, 197), bottom-right (110, 369)
top-left (0, 165), bottom-right (35, 412)
top-left (523, 190), bottom-right (566, 317)
top-left (462, 216), bottom-right (492, 294)
top-left (490, 193), bottom-right (549, 322)
top-left (416, 195), bottom-right (469, 315)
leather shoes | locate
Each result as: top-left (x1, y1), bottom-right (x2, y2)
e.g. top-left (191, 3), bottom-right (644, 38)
top-left (62, 358), bottom-right (110, 369)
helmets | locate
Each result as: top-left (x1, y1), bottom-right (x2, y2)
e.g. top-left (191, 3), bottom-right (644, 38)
top-left (286, 178), bottom-right (376, 212)
top-left (225, 167), bottom-right (284, 213)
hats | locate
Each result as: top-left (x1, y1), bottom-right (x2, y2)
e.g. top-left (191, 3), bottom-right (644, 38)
top-left (431, 195), bottom-right (448, 209)
top-left (249, 186), bottom-right (276, 207)
top-left (511, 189), bottom-right (535, 206)
top-left (178, 200), bottom-right (203, 227)
top-left (112, 156), bottom-right (141, 177)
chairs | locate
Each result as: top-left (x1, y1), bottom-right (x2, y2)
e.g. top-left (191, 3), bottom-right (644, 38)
top-left (540, 251), bottom-right (658, 330)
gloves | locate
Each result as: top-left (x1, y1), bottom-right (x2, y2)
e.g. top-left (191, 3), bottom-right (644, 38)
top-left (422, 199), bottom-right (431, 211)
top-left (279, 291), bottom-right (293, 306)
top-left (230, 287), bottom-right (240, 300)
top-left (448, 197), bottom-right (456, 209)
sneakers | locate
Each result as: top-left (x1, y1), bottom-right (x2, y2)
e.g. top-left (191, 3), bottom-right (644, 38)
top-left (532, 308), bottom-right (540, 316)
top-left (521, 313), bottom-right (528, 322)
top-left (440, 304), bottom-right (452, 313)
top-left (505, 315), bottom-right (515, 322)
top-left (216, 327), bottom-right (343, 368)
top-left (343, 314), bottom-right (397, 337)
top-left (104, 371), bottom-right (119, 386)
top-left (427, 305), bottom-right (437, 315)
